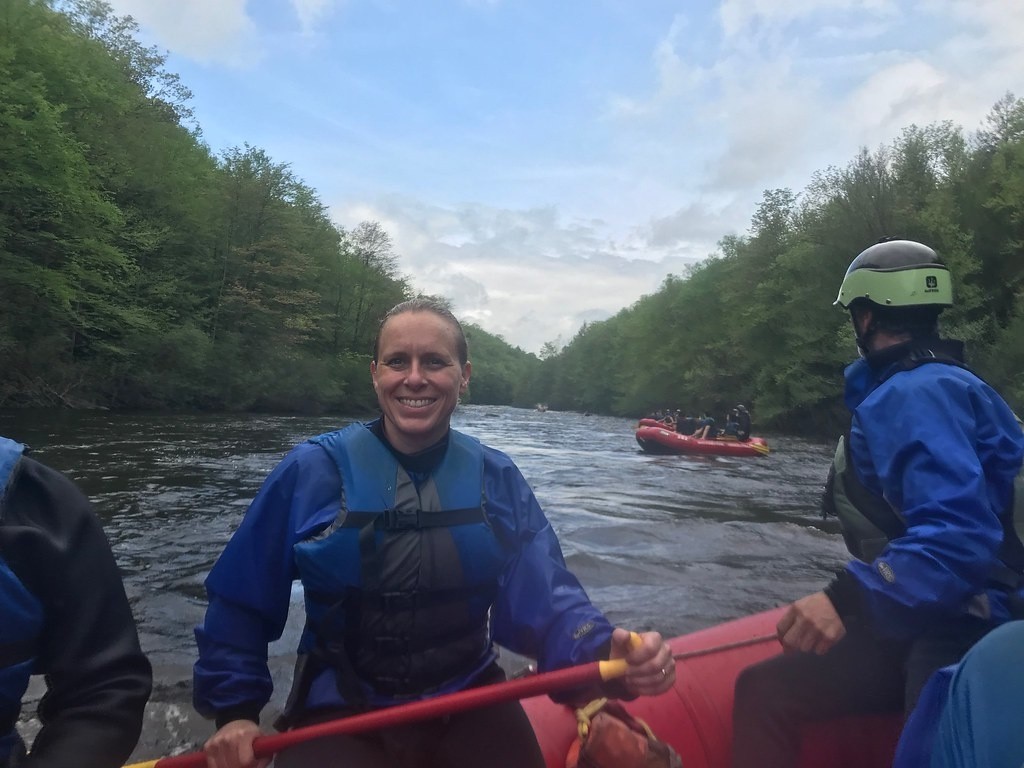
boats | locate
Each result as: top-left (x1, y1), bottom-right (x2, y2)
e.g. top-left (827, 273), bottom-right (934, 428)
top-left (635, 418), bottom-right (770, 457)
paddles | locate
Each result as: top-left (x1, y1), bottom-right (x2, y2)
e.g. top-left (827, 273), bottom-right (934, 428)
top-left (119, 629), bottom-right (648, 767)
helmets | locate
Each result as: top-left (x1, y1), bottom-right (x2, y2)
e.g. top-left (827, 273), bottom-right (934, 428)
top-left (833, 240), bottom-right (954, 308)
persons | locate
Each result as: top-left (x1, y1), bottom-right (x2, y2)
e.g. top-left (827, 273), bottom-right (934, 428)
top-left (655, 404), bottom-right (750, 442)
top-left (732, 236), bottom-right (1024, 768)
top-left (0, 436), bottom-right (153, 768)
top-left (192, 298), bottom-right (675, 768)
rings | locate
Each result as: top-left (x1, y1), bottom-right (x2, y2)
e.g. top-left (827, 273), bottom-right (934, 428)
top-left (661, 668), bottom-right (666, 677)
